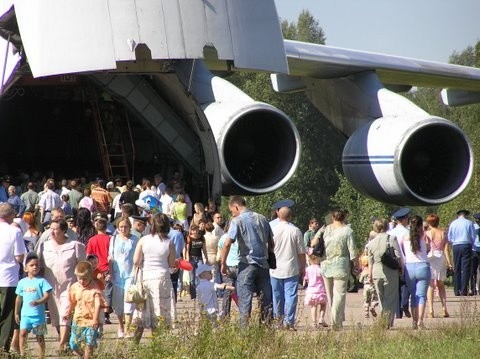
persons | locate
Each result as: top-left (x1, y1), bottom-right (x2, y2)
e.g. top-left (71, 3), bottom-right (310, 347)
top-left (62, 261), bottom-right (102, 359)
top-left (14, 252), bottom-right (53, 358)
top-left (0, 202), bottom-right (26, 352)
top-left (0, 170), bottom-right (480, 344)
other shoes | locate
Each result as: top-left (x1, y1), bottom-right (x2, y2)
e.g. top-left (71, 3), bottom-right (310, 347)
top-left (369, 307), bottom-right (377, 317)
top-left (400, 305), bottom-right (411, 319)
top-left (412, 321), bottom-right (417, 329)
top-left (364, 313), bottom-right (369, 318)
top-left (319, 319), bottom-right (328, 327)
top-left (417, 322), bottom-right (426, 329)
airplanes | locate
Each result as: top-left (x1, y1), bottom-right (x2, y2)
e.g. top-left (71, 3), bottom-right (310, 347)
top-left (0, 0), bottom-right (480, 206)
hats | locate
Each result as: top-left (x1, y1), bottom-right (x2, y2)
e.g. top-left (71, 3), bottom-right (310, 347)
top-left (132, 215), bottom-right (149, 226)
top-left (22, 252), bottom-right (38, 268)
top-left (91, 211), bottom-right (109, 221)
top-left (473, 213), bottom-right (480, 221)
top-left (393, 208), bottom-right (411, 220)
top-left (196, 264), bottom-right (212, 276)
top-left (273, 198), bottom-right (295, 210)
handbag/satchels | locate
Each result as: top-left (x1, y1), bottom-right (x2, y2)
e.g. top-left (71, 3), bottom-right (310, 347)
top-left (381, 234), bottom-right (399, 270)
top-left (426, 231), bottom-right (443, 262)
top-left (125, 265), bottom-right (149, 303)
top-left (311, 231), bottom-right (325, 257)
